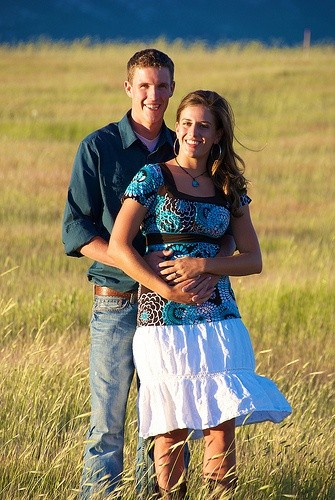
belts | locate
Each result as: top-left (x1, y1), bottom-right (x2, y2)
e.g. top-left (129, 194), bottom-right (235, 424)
top-left (94, 284), bottom-right (139, 300)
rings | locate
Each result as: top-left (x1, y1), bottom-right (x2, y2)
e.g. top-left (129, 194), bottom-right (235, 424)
top-left (175, 272), bottom-right (178, 278)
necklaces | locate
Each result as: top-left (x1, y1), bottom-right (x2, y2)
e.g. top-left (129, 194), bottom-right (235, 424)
top-left (174, 157), bottom-right (207, 187)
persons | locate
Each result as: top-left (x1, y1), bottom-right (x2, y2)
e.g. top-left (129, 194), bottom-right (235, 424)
top-left (105, 89), bottom-right (291, 500)
top-left (62, 48), bottom-right (236, 500)
